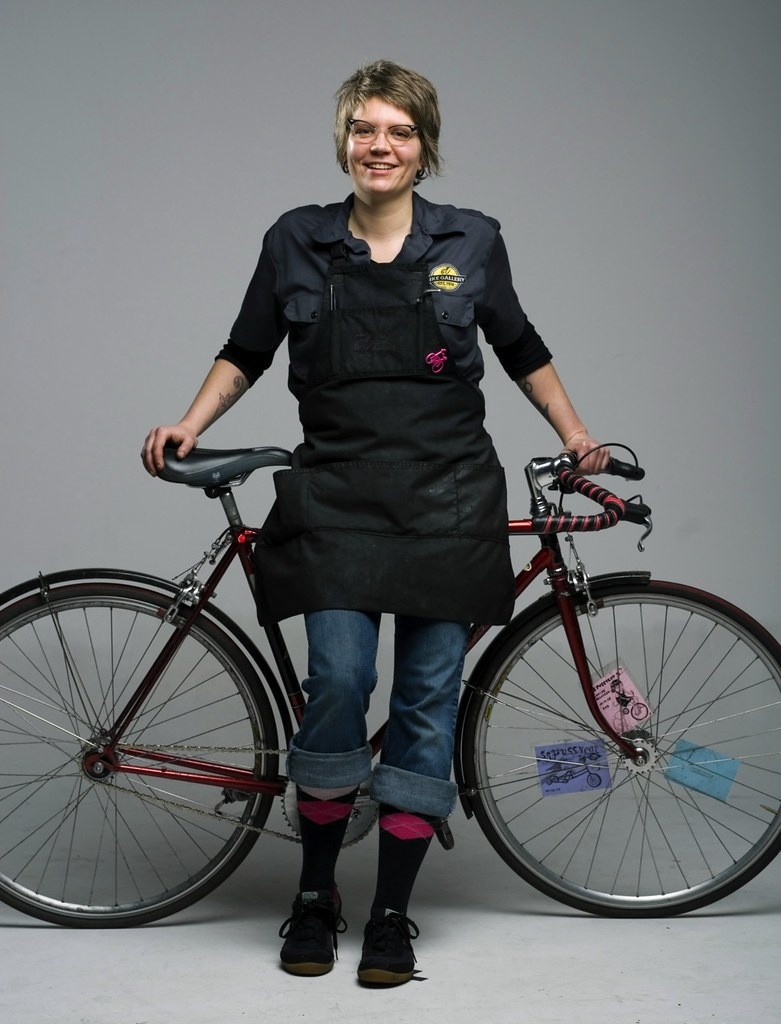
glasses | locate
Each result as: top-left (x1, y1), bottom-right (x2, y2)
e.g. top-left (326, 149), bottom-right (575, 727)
top-left (348, 119), bottom-right (418, 146)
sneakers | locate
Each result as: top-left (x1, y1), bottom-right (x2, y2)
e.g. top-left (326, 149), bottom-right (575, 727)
top-left (278, 880), bottom-right (347, 975)
top-left (357, 907), bottom-right (419, 988)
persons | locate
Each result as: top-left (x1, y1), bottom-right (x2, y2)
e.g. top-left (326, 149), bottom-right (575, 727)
top-left (141, 59), bottom-right (616, 993)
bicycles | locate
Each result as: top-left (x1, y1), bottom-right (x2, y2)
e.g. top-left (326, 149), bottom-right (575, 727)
top-left (0, 444), bottom-right (781, 929)
